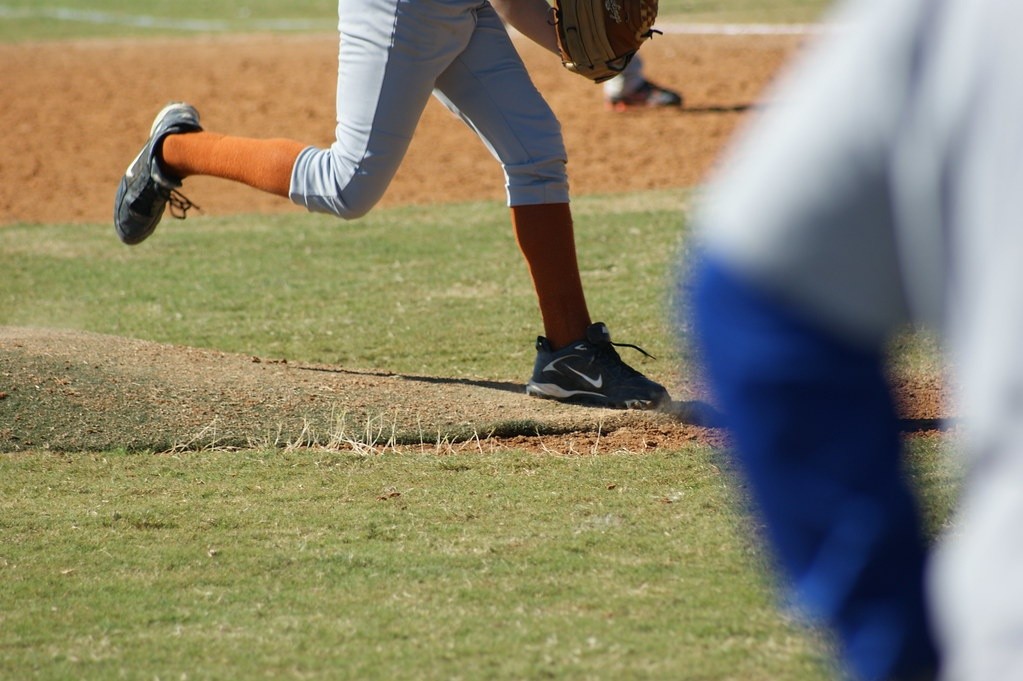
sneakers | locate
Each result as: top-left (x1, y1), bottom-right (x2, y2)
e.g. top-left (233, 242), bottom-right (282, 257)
top-left (113, 104), bottom-right (206, 246)
top-left (528, 322), bottom-right (672, 410)
top-left (611, 83), bottom-right (681, 111)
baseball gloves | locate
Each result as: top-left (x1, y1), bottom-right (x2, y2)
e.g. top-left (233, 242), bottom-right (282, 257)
top-left (552, 0), bottom-right (659, 85)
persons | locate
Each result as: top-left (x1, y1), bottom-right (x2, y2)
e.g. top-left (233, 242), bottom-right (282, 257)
top-left (676, 0), bottom-right (1023, 681)
top-left (114, 0), bottom-right (671, 409)
top-left (602, 51), bottom-right (681, 110)
